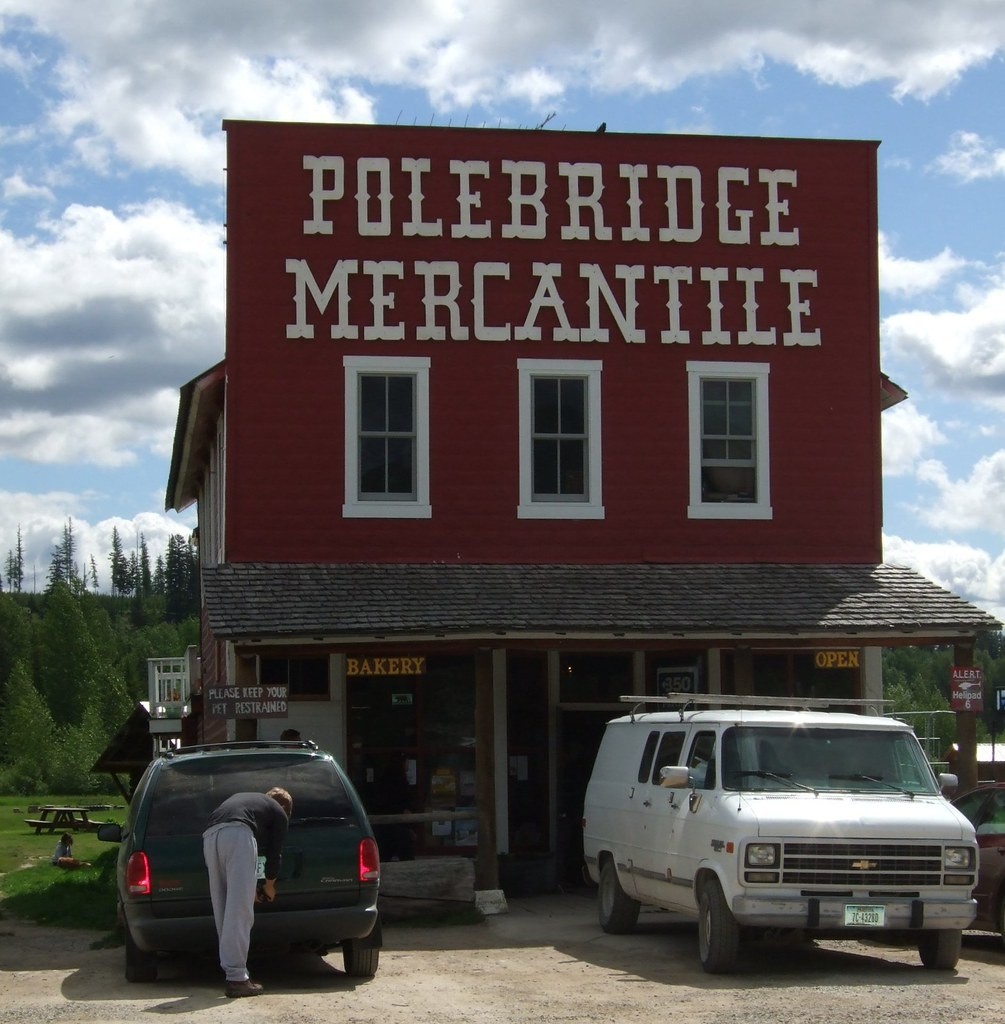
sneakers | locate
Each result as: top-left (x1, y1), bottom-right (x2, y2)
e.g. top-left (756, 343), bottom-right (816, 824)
top-left (225, 980), bottom-right (262, 998)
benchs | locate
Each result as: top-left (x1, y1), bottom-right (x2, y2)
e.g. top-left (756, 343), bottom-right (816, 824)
top-left (24, 819), bottom-right (52, 824)
top-left (75, 818), bottom-right (104, 824)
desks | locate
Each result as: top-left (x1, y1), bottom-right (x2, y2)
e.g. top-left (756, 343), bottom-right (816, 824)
top-left (29, 807), bottom-right (99, 835)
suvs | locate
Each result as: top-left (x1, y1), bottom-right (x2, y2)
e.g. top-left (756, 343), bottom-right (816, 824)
top-left (97, 739), bottom-right (383, 983)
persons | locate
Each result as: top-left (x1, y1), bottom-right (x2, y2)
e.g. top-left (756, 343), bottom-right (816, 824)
top-left (203, 786), bottom-right (293, 997)
top-left (364, 752), bottom-right (417, 862)
top-left (280, 729), bottom-right (302, 749)
top-left (52, 832), bottom-right (81, 870)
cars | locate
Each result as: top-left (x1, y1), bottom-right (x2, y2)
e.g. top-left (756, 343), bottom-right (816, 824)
top-left (939, 782), bottom-right (1005, 940)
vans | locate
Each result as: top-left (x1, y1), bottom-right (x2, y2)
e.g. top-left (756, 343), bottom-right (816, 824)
top-left (581, 692), bottom-right (979, 974)
